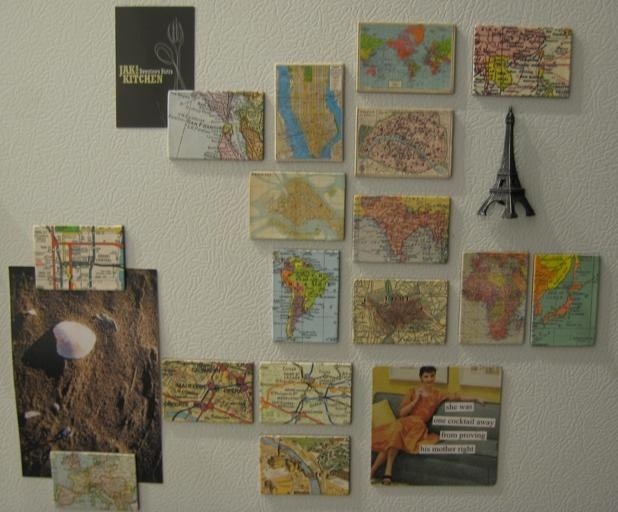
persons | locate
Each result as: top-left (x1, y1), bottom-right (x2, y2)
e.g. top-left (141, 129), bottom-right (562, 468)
top-left (370, 365), bottom-right (486, 487)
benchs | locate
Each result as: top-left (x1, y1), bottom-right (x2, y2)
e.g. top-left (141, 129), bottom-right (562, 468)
top-left (372, 393), bottom-right (501, 486)
